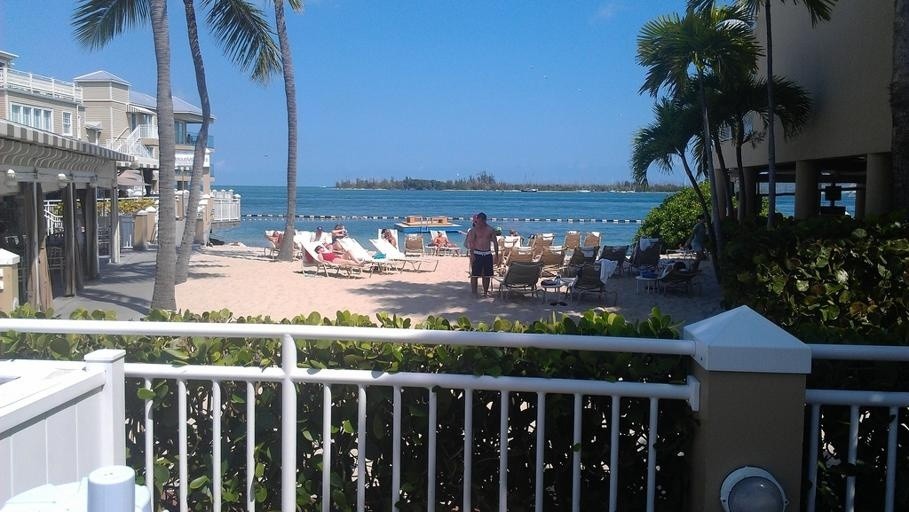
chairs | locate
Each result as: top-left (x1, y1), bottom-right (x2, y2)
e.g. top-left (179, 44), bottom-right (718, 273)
top-left (489, 230), bottom-right (702, 304)
top-left (265, 228), bottom-right (460, 279)
top-left (4, 216), bottom-right (118, 315)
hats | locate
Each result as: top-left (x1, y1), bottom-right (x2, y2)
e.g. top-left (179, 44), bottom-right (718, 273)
top-left (317, 226), bottom-right (326, 232)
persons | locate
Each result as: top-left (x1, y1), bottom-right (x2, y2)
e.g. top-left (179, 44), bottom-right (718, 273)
top-left (309, 224), bottom-right (397, 267)
top-left (468, 213), bottom-right (498, 299)
top-left (433, 231), bottom-right (457, 248)
top-left (490, 227), bottom-right (522, 266)
top-left (685, 213), bottom-right (707, 270)
top-left (273, 231), bottom-right (283, 249)
top-left (463, 214), bottom-right (479, 286)
